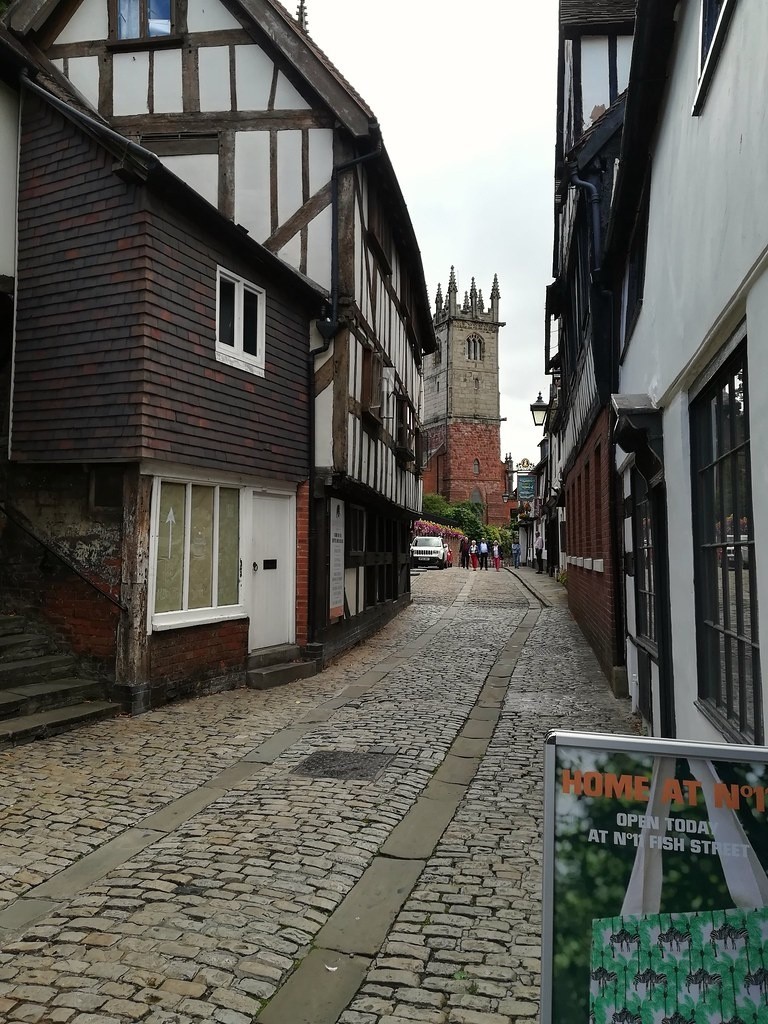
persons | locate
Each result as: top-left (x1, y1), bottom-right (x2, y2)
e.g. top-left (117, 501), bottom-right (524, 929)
top-left (534, 531), bottom-right (544, 574)
top-left (511, 538), bottom-right (522, 569)
top-left (459, 535), bottom-right (502, 572)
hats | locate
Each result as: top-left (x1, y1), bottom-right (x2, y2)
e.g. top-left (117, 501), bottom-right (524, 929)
top-left (493, 540), bottom-right (499, 545)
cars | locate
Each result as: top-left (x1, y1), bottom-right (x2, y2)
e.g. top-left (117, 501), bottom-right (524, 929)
top-left (443, 542), bottom-right (454, 567)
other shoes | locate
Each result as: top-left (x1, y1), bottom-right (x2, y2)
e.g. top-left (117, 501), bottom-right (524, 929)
top-left (485, 568), bottom-right (488, 571)
top-left (496, 569), bottom-right (500, 572)
top-left (480, 567), bottom-right (482, 570)
top-left (473, 569), bottom-right (476, 571)
top-left (535, 571), bottom-right (542, 574)
top-left (517, 566), bottom-right (519, 569)
top-left (466, 566), bottom-right (468, 569)
top-left (463, 564), bottom-right (465, 568)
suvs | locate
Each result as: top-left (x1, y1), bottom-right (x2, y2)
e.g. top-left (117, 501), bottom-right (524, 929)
top-left (410, 536), bottom-right (449, 570)
